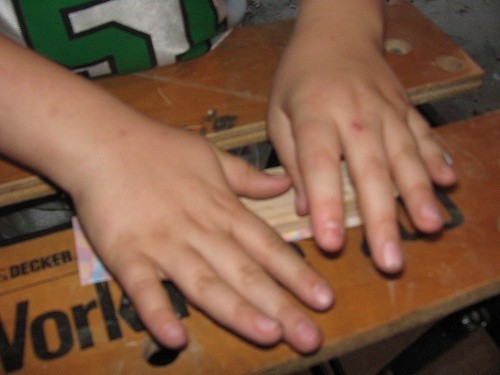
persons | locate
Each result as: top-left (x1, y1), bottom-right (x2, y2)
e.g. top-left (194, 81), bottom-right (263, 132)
top-left (0, 0), bottom-right (458, 357)
